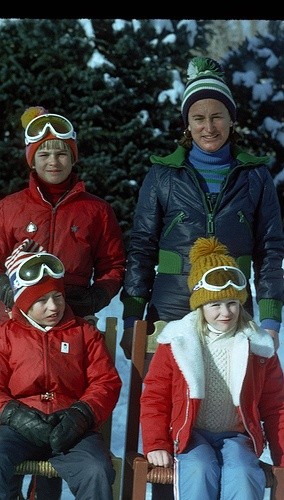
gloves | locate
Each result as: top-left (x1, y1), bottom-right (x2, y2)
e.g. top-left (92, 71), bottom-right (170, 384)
top-left (68, 279), bottom-right (111, 316)
top-left (2, 400), bottom-right (53, 448)
top-left (47, 401), bottom-right (94, 452)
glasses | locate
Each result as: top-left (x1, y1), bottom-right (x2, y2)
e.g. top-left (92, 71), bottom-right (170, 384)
top-left (13, 253), bottom-right (66, 288)
top-left (190, 265), bottom-right (248, 296)
top-left (24, 113), bottom-right (77, 145)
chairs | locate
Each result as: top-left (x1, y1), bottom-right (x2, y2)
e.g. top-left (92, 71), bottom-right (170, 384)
top-left (10, 317), bottom-right (122, 500)
top-left (121, 320), bottom-right (284, 500)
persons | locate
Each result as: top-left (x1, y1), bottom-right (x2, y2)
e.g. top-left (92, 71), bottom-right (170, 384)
top-left (0, 106), bottom-right (126, 500)
top-left (141, 237), bottom-right (284, 500)
top-left (0, 238), bottom-right (123, 500)
top-left (118, 57), bottom-right (284, 500)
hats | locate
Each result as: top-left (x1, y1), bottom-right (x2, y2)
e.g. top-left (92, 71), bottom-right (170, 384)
top-left (187, 236), bottom-right (247, 310)
top-left (6, 238), bottom-right (66, 312)
top-left (180, 57), bottom-right (236, 127)
top-left (21, 106), bottom-right (78, 169)
